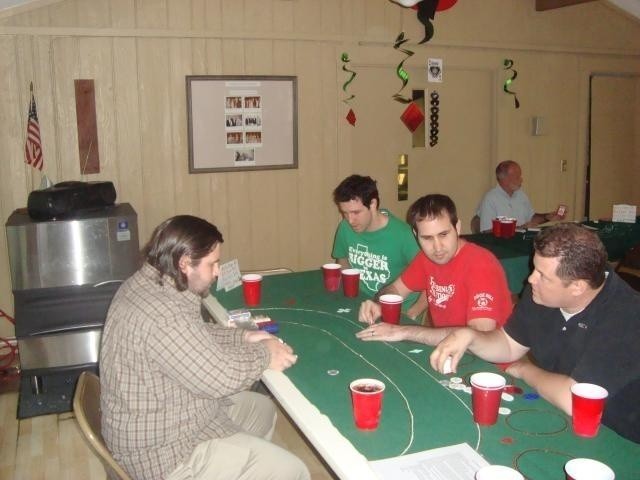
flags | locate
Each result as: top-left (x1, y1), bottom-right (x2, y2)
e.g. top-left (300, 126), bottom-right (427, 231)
top-left (23, 93), bottom-right (44, 171)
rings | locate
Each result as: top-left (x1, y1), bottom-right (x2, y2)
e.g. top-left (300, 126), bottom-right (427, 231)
top-left (371, 331), bottom-right (374, 336)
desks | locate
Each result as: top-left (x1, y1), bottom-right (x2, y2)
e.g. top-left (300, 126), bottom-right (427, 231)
top-left (460, 218), bottom-right (640, 293)
top-left (200, 267), bottom-right (639, 479)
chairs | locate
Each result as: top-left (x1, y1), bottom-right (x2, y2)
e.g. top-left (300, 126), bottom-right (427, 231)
top-left (70, 371), bottom-right (136, 479)
top-left (421, 289), bottom-right (521, 328)
top-left (469, 215), bottom-right (482, 234)
top-left (613, 242), bottom-right (639, 292)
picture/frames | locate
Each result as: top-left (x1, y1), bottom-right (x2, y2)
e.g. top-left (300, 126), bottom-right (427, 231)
top-left (184, 74), bottom-right (301, 174)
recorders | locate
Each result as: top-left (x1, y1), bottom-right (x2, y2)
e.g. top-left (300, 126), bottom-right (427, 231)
top-left (26, 179), bottom-right (118, 220)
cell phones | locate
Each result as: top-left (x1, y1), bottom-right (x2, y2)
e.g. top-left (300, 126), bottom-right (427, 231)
top-left (556, 204), bottom-right (567, 216)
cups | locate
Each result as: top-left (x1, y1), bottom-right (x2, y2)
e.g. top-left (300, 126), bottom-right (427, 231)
top-left (564, 458), bottom-right (616, 480)
top-left (321, 263), bottom-right (343, 292)
top-left (241, 273), bottom-right (264, 305)
top-left (569, 382), bottom-right (610, 439)
top-left (349, 377), bottom-right (386, 431)
top-left (341, 267), bottom-right (360, 298)
top-left (492, 215), bottom-right (517, 239)
top-left (468, 372), bottom-right (507, 426)
top-left (378, 294), bottom-right (404, 325)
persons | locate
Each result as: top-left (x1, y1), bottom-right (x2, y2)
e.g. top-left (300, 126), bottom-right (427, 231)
top-left (356, 194), bottom-right (519, 373)
top-left (97, 212), bottom-right (311, 480)
top-left (477, 160), bottom-right (567, 234)
top-left (330, 174), bottom-right (428, 327)
top-left (429, 221), bottom-right (640, 445)
